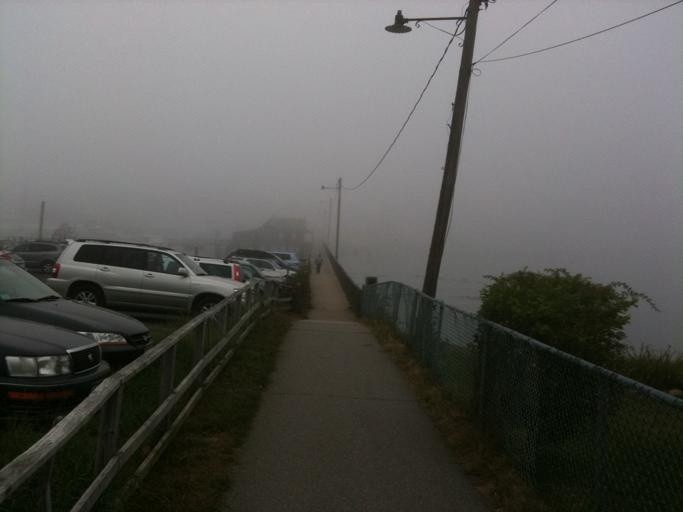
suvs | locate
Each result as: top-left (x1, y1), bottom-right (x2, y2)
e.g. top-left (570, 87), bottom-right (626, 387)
top-left (46, 239), bottom-right (246, 328)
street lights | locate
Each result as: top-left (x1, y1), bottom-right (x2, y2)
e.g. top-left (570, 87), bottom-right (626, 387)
top-left (321, 185), bottom-right (342, 264)
top-left (384, 9), bottom-right (480, 361)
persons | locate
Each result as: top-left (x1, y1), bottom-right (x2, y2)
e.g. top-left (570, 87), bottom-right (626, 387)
top-left (313, 253), bottom-right (322, 273)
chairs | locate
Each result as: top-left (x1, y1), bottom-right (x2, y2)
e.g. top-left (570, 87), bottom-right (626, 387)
top-left (164, 261), bottom-right (178, 275)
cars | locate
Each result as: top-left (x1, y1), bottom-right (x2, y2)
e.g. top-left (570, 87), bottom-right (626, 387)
top-left (0, 256), bottom-right (155, 422)
top-left (163, 248), bottom-right (300, 283)
top-left (0, 240), bottom-right (67, 274)
